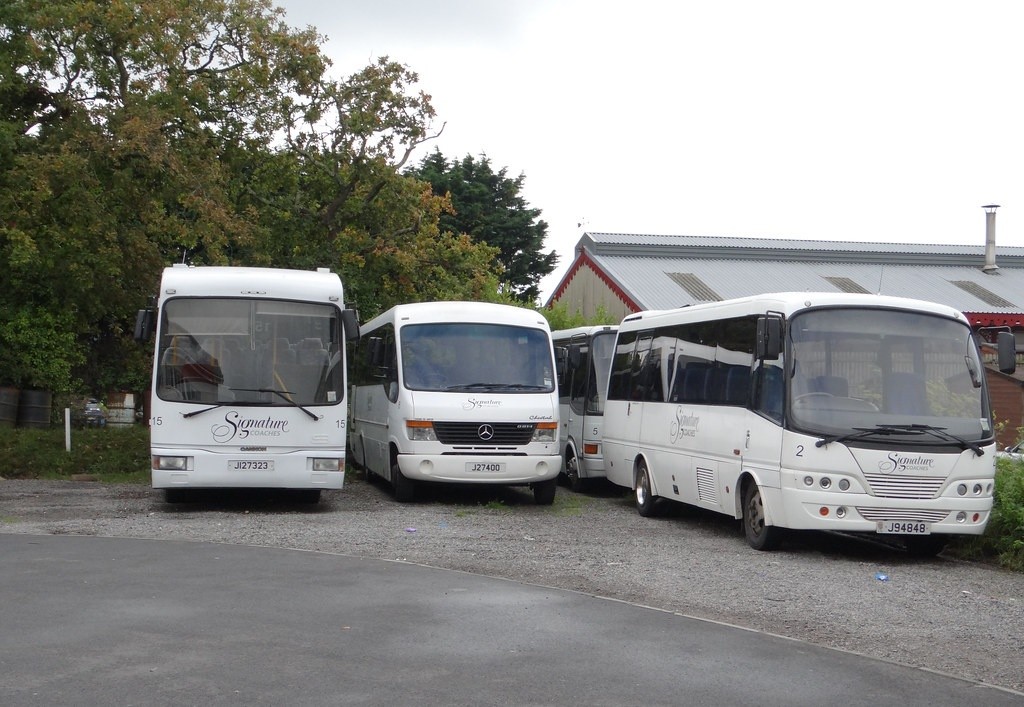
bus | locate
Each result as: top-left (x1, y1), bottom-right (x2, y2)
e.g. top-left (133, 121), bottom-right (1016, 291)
top-left (601, 289), bottom-right (1017, 550)
top-left (346, 300), bottom-right (569, 505)
top-left (131, 262), bottom-right (362, 504)
top-left (546, 323), bottom-right (620, 494)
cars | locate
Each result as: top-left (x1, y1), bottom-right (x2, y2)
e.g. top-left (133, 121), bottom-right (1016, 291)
top-left (85, 397), bottom-right (108, 429)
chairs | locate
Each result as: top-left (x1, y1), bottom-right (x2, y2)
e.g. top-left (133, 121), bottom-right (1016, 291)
top-left (882, 371), bottom-right (930, 416)
top-left (162, 337), bottom-right (331, 370)
top-left (656, 358), bottom-right (850, 407)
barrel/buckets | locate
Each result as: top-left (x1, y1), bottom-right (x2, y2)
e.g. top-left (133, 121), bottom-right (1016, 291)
top-left (142, 389), bottom-right (151, 428)
top-left (106, 392), bottom-right (137, 430)
top-left (0, 386), bottom-right (20, 429)
top-left (20, 389), bottom-right (53, 431)
